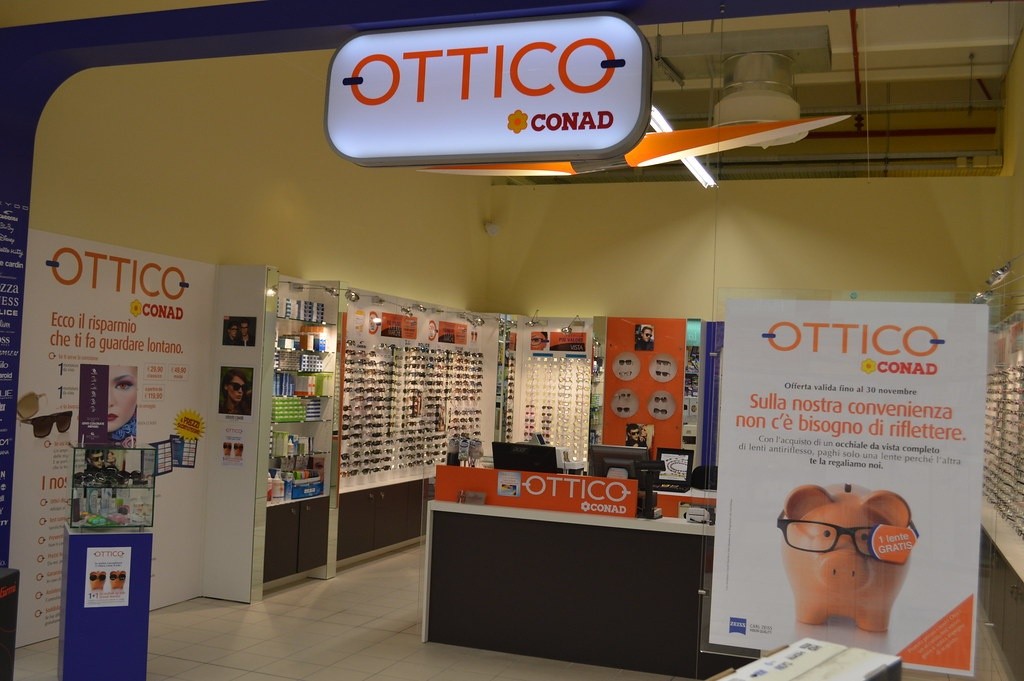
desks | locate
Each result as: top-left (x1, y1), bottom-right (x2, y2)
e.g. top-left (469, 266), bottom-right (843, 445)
top-left (422, 494), bottom-right (716, 681)
top-left (708, 638), bottom-right (902, 681)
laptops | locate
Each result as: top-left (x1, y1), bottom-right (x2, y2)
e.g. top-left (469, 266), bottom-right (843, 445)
top-left (651, 447), bottom-right (695, 493)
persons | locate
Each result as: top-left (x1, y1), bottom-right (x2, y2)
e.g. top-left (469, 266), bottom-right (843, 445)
top-left (219, 368), bottom-right (251, 415)
top-left (84, 449), bottom-right (124, 478)
top-left (222, 319), bottom-right (256, 347)
top-left (107, 366), bottom-right (138, 448)
top-left (634, 326), bottom-right (654, 351)
top-left (626, 424), bottom-right (649, 448)
top-left (530, 331), bottom-right (548, 350)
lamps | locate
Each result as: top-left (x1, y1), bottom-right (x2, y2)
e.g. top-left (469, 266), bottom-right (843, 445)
top-left (650, 74), bottom-right (718, 190)
top-left (280, 280), bottom-right (339, 297)
top-left (372, 296), bottom-right (413, 318)
top-left (432, 308), bottom-right (484, 327)
top-left (298, 286), bottom-right (360, 302)
top-left (414, 304), bottom-right (427, 312)
top-left (983, 251), bottom-right (1022, 286)
top-left (970, 290), bottom-right (1013, 305)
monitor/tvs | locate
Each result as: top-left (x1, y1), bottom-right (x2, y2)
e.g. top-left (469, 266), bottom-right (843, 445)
top-left (590, 445), bottom-right (649, 492)
top-left (492, 442), bottom-right (557, 474)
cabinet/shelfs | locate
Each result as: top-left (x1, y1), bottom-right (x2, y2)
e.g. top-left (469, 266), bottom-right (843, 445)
top-left (262, 481), bottom-right (423, 593)
top-left (200, 264), bottom-right (329, 603)
top-left (68, 439), bottom-right (157, 532)
top-left (685, 372), bottom-right (698, 397)
top-left (588, 358), bottom-right (604, 462)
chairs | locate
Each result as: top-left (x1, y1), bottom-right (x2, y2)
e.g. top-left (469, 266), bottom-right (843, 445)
top-left (683, 466), bottom-right (716, 525)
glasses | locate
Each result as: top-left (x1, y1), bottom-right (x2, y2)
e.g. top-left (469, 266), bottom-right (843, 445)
top-left (656, 359), bottom-right (671, 366)
top-left (619, 393), bottom-right (631, 398)
top-left (655, 370), bottom-right (670, 378)
top-left (234, 444), bottom-right (243, 449)
top-left (229, 382), bottom-right (248, 392)
top-left (654, 408), bottom-right (667, 415)
top-left (89, 575), bottom-right (106, 581)
top-left (523, 356), bottom-right (590, 461)
top-left (340, 338), bottom-right (482, 478)
top-left (776, 510), bottom-right (919, 561)
top-left (619, 360), bottom-right (631, 366)
top-left (629, 431), bottom-right (639, 435)
top-left (75, 466), bottom-right (150, 487)
top-left (223, 444), bottom-right (231, 449)
top-left (644, 333), bottom-right (652, 337)
top-left (507, 353), bottom-right (515, 441)
top-left (91, 456), bottom-right (104, 462)
top-left (617, 407), bottom-right (630, 412)
top-left (618, 370), bottom-right (632, 376)
top-left (654, 396), bottom-right (666, 403)
top-left (110, 573), bottom-right (126, 580)
top-left (982, 366), bottom-right (1023, 540)
top-left (20, 410), bottom-right (73, 437)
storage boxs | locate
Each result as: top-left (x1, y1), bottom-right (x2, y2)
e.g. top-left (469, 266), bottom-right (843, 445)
top-left (292, 481), bottom-right (322, 500)
top-left (305, 399), bottom-right (321, 421)
top-left (286, 335), bottom-right (314, 352)
top-left (278, 339), bottom-right (294, 349)
top-left (684, 396), bottom-right (690, 416)
top-left (298, 372), bottom-right (333, 397)
top-left (272, 395), bottom-right (305, 423)
top-left (690, 397), bottom-right (698, 416)
top-left (301, 326), bottom-right (324, 333)
top-left (314, 338), bottom-right (326, 351)
